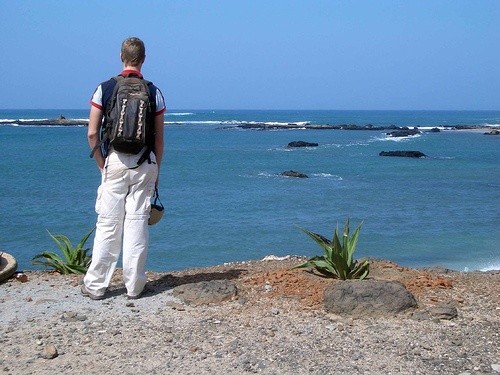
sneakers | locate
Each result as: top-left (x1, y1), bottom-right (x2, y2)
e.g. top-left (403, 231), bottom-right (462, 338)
top-left (126, 284), bottom-right (146, 299)
top-left (81, 284), bottom-right (106, 300)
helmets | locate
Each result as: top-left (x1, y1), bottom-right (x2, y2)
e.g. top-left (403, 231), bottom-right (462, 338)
top-left (148, 204), bottom-right (164, 225)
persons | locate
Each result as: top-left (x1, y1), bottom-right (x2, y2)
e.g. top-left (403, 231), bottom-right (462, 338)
top-left (80, 36), bottom-right (168, 301)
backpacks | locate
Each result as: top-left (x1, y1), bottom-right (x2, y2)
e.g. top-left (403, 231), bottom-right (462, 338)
top-left (104, 78), bottom-right (150, 155)
